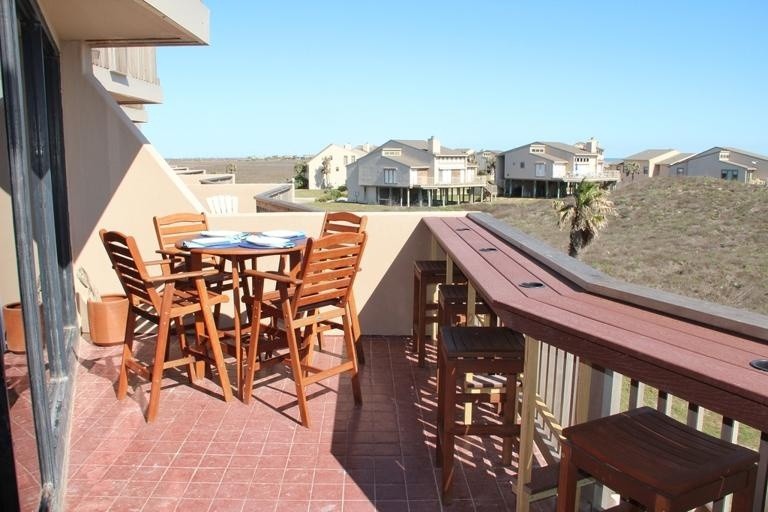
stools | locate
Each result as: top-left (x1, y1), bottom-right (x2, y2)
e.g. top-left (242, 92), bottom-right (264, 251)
top-left (436, 284), bottom-right (504, 418)
top-left (434, 326), bottom-right (526, 506)
top-left (555, 405), bottom-right (759, 511)
top-left (413, 261), bottom-right (469, 367)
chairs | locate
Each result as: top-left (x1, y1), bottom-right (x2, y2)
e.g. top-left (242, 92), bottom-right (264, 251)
top-left (243, 233), bottom-right (366, 427)
top-left (99, 227), bottom-right (233, 424)
top-left (263, 210), bottom-right (366, 379)
top-left (152, 212), bottom-right (261, 381)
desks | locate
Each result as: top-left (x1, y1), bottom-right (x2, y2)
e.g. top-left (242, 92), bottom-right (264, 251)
top-left (174, 229), bottom-right (309, 399)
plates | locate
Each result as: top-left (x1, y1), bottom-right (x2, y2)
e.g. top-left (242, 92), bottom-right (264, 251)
top-left (191, 230), bottom-right (306, 247)
